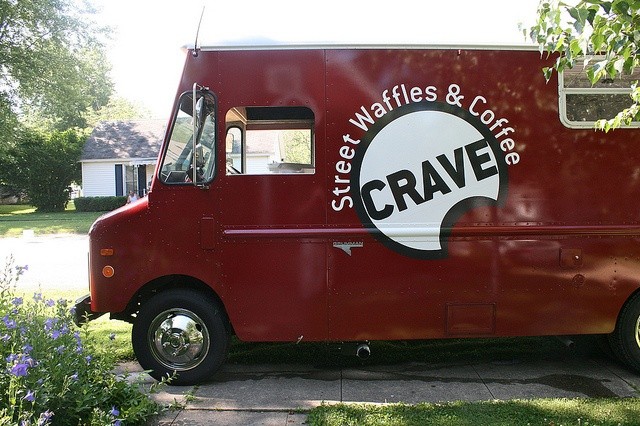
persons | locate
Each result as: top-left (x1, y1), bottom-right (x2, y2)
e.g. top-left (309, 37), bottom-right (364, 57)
top-left (126, 189), bottom-right (140, 204)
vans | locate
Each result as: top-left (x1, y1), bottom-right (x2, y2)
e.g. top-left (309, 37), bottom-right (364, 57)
top-left (72, 5), bottom-right (640, 386)
